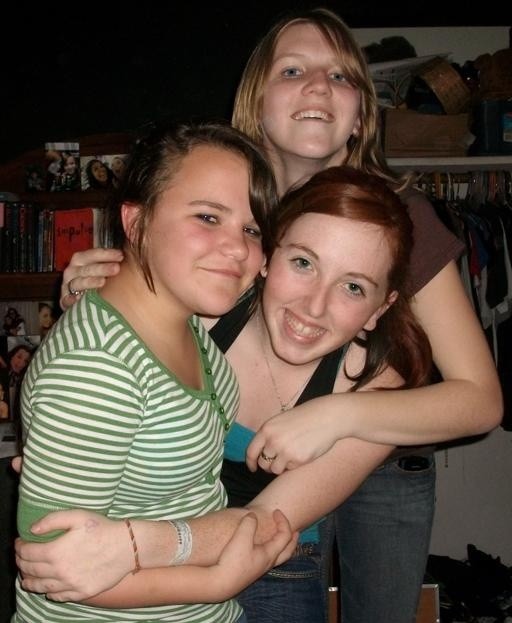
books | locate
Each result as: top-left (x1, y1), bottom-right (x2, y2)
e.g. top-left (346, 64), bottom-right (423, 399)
top-left (0, 199), bottom-right (115, 273)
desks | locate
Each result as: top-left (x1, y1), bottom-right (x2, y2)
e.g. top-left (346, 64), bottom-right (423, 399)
top-left (0, 435), bottom-right (24, 621)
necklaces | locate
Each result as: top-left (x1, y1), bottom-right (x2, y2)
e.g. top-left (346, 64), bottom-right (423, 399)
top-left (261, 327), bottom-right (317, 413)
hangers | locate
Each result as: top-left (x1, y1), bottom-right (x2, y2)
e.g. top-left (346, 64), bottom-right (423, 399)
top-left (403, 163), bottom-right (511, 238)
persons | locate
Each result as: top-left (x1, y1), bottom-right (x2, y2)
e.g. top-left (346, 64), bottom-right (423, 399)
top-left (231, 5), bottom-right (504, 623)
top-left (8, 122), bottom-right (300, 622)
top-left (29, 150), bottom-right (61, 193)
top-left (111, 157), bottom-right (125, 188)
top-left (1, 302), bottom-right (53, 387)
top-left (84, 159), bottom-right (116, 193)
top-left (14, 165), bottom-right (433, 622)
top-left (60, 155), bottom-right (81, 191)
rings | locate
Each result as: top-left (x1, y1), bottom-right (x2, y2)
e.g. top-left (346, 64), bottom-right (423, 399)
top-left (260, 447), bottom-right (276, 462)
top-left (67, 280), bottom-right (80, 295)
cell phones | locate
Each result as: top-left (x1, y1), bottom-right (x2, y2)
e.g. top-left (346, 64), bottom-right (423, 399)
top-left (398, 455), bottom-right (429, 471)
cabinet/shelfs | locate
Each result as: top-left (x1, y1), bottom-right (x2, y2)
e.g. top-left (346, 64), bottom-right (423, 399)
top-left (0, 127), bottom-right (152, 305)
top-left (344, 21), bottom-right (510, 622)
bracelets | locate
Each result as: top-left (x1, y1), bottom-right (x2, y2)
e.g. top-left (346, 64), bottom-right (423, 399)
top-left (166, 519), bottom-right (193, 567)
top-left (124, 518), bottom-right (141, 572)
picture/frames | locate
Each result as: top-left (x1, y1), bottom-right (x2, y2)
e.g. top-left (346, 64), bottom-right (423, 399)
top-left (325, 580), bottom-right (443, 622)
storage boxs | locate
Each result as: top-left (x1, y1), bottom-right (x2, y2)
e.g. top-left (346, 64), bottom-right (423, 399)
top-left (467, 90), bottom-right (512, 156)
top-left (376, 103), bottom-right (472, 160)
